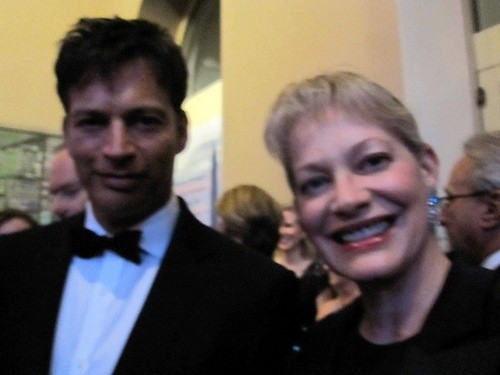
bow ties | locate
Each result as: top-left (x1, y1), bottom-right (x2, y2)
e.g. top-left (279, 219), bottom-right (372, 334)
top-left (75, 227), bottom-right (143, 265)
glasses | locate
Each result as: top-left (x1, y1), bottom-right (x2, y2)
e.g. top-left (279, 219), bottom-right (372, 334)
top-left (438, 192), bottom-right (473, 204)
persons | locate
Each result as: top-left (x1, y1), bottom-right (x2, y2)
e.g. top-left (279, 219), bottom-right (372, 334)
top-left (232, 71), bottom-right (500, 375)
top-left (274, 203), bottom-right (337, 302)
top-left (213, 185), bottom-right (282, 259)
top-left (47, 141), bottom-right (90, 220)
top-left (0, 18), bottom-right (319, 375)
top-left (433, 130), bottom-right (500, 273)
top-left (0, 209), bottom-right (38, 233)
top-left (314, 250), bottom-right (362, 321)
